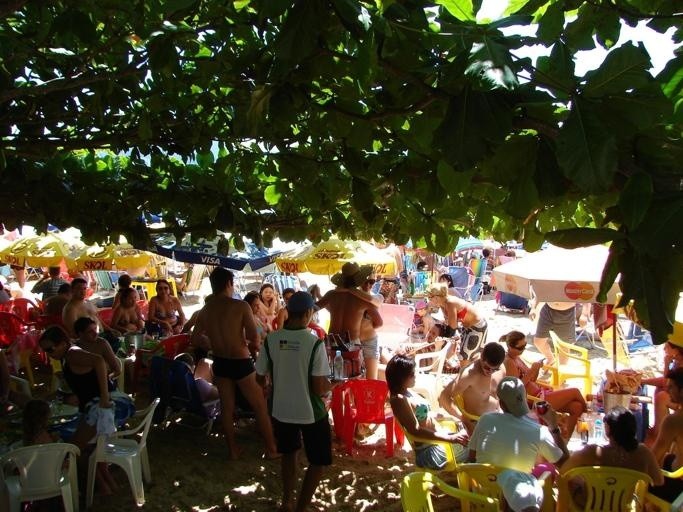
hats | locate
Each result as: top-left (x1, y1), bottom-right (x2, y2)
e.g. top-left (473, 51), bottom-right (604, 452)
top-left (496, 469), bottom-right (544, 511)
top-left (287, 290), bottom-right (320, 311)
top-left (331, 262), bottom-right (373, 288)
top-left (497, 376), bottom-right (530, 417)
top-left (415, 302), bottom-right (427, 309)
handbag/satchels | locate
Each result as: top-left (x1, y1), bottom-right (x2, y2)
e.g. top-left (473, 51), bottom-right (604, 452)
top-left (605, 370), bottom-right (644, 394)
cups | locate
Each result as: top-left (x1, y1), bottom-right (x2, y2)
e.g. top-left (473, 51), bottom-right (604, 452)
top-left (580, 429), bottom-right (589, 444)
top-left (536, 400), bottom-right (550, 426)
top-left (576, 419), bottom-right (587, 432)
top-left (151, 332), bottom-right (158, 340)
top-left (342, 358), bottom-right (360, 378)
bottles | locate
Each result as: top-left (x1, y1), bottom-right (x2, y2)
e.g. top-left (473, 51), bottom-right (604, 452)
top-left (593, 419), bottom-right (602, 440)
top-left (48, 400), bottom-right (56, 416)
top-left (56, 402), bottom-right (63, 413)
top-left (333, 350), bottom-right (343, 378)
top-left (408, 278), bottom-right (414, 295)
top-left (327, 329), bottom-right (351, 352)
top-left (128, 344), bottom-right (136, 356)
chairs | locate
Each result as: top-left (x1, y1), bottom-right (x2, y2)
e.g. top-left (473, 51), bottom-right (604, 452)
top-left (400, 260), bottom-right (682, 512)
top-left (271, 314), bottom-right (407, 460)
top-left (0, 258), bottom-right (218, 511)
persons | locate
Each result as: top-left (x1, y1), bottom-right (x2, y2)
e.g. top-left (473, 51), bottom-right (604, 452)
top-left (0, 248), bottom-right (683, 512)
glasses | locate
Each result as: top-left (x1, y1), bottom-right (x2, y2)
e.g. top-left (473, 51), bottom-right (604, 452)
top-left (515, 342), bottom-right (527, 352)
top-left (366, 279), bottom-right (375, 285)
top-left (42, 343), bottom-right (60, 354)
top-left (483, 365), bottom-right (500, 372)
